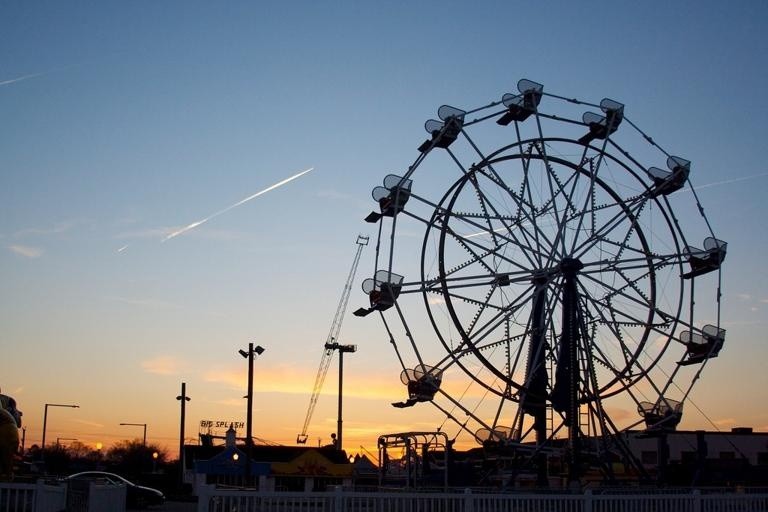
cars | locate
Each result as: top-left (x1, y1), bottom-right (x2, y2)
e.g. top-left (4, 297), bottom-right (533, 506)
top-left (57, 470), bottom-right (164, 505)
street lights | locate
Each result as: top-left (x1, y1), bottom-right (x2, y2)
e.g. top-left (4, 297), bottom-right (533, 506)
top-left (238, 343), bottom-right (267, 444)
top-left (349, 457), bottom-right (355, 490)
top-left (95, 442), bottom-right (103, 461)
top-left (56, 438), bottom-right (77, 448)
top-left (176, 382), bottom-right (191, 463)
top-left (153, 451), bottom-right (159, 473)
top-left (324, 341), bottom-right (358, 448)
top-left (119, 424), bottom-right (147, 446)
top-left (40, 404), bottom-right (80, 449)
